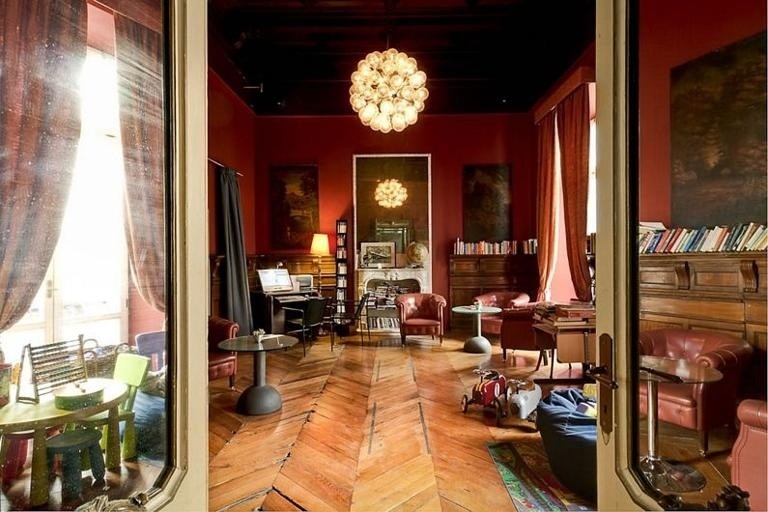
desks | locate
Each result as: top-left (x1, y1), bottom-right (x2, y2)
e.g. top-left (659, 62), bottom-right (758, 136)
top-left (635, 354), bottom-right (724, 495)
top-left (217, 333), bottom-right (300, 415)
top-left (250, 290), bottom-right (323, 334)
top-left (0, 377), bottom-right (130, 507)
top-left (530, 320), bottom-right (596, 379)
top-left (452, 304), bottom-right (503, 353)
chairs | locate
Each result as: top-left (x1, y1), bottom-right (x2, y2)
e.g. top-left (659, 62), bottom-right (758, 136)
top-left (498, 302), bottom-right (556, 366)
top-left (62, 352), bottom-right (152, 471)
top-left (394, 292), bottom-right (447, 345)
top-left (280, 294), bottom-right (334, 357)
top-left (728, 397), bottom-right (768, 512)
top-left (135, 331), bottom-right (166, 372)
top-left (208, 315), bottom-right (241, 389)
top-left (329, 292), bottom-right (371, 352)
top-left (471, 291), bottom-right (531, 336)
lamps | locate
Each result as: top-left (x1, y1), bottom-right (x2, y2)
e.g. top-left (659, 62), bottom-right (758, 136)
top-left (373, 176), bottom-right (408, 209)
top-left (309, 232), bottom-right (331, 336)
top-left (349, 37), bottom-right (430, 135)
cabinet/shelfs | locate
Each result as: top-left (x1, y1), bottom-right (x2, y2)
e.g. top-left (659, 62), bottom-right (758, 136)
top-left (639, 251), bottom-right (768, 390)
top-left (448, 253), bottom-right (538, 331)
top-left (354, 270), bottom-right (433, 337)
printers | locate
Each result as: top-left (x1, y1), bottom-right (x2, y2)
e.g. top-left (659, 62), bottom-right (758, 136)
top-left (290, 275), bottom-right (321, 292)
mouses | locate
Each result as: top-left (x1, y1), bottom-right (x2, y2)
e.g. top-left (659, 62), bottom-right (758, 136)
top-left (305, 294), bottom-right (309, 298)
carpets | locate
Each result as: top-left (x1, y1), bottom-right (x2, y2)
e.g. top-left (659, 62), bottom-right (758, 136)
top-left (484, 435), bottom-right (732, 512)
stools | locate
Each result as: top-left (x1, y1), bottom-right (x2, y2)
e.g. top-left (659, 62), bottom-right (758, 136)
top-left (44, 429), bottom-right (107, 505)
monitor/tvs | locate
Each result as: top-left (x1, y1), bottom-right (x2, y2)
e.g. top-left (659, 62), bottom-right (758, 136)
top-left (257, 268), bottom-right (294, 292)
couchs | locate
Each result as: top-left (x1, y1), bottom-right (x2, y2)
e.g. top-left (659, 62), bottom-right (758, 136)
top-left (637, 326), bottom-right (754, 457)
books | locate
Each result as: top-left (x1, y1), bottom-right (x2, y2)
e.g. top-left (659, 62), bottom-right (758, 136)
top-left (585, 232), bottom-right (598, 253)
top-left (366, 284), bottom-right (414, 329)
top-left (639, 218), bottom-right (768, 252)
top-left (532, 294), bottom-right (595, 329)
top-left (450, 237), bottom-right (538, 258)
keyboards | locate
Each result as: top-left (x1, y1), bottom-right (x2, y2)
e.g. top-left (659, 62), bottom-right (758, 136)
top-left (275, 295), bottom-right (305, 300)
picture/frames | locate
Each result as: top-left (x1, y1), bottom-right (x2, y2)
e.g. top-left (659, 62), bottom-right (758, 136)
top-left (360, 241), bottom-right (396, 269)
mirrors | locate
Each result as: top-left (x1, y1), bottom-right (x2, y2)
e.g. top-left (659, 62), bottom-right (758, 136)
top-left (352, 153), bottom-right (433, 269)
